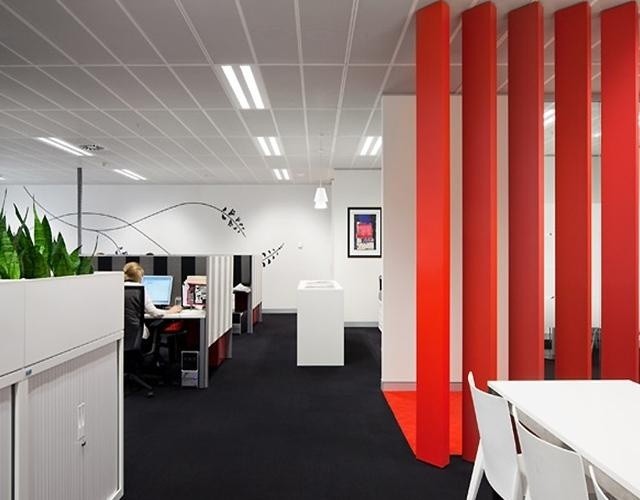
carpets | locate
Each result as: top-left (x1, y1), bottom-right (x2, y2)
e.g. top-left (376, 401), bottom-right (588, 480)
top-left (384, 390), bottom-right (464, 458)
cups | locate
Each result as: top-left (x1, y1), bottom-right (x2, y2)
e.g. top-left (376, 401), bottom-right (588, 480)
top-left (174, 296), bottom-right (182, 313)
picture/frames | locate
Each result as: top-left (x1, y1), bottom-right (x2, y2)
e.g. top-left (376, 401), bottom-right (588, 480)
top-left (347, 206), bottom-right (382, 258)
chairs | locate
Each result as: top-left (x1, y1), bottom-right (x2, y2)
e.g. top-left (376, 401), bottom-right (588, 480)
top-left (589, 465), bottom-right (611, 500)
top-left (123, 284), bottom-right (165, 398)
top-left (465, 370), bottom-right (530, 500)
top-left (510, 404), bottom-right (608, 500)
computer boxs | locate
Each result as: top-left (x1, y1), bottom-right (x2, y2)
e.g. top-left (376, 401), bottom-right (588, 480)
top-left (232, 311), bottom-right (247, 334)
top-left (181, 351), bottom-right (199, 388)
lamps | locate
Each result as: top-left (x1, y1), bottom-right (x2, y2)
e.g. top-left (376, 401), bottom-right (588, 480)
top-left (314, 178), bottom-right (328, 210)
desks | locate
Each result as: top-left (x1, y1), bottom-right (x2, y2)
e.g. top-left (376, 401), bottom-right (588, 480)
top-left (486, 379), bottom-right (640, 500)
top-left (143, 308), bottom-right (206, 390)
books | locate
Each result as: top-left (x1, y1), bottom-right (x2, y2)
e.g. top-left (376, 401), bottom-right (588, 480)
top-left (181, 275), bottom-right (208, 310)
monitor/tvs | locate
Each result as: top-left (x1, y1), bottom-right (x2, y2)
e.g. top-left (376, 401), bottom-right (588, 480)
top-left (141, 276), bottom-right (173, 309)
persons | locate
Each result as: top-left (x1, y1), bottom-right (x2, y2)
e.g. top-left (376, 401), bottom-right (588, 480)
top-left (124, 261), bottom-right (182, 388)
